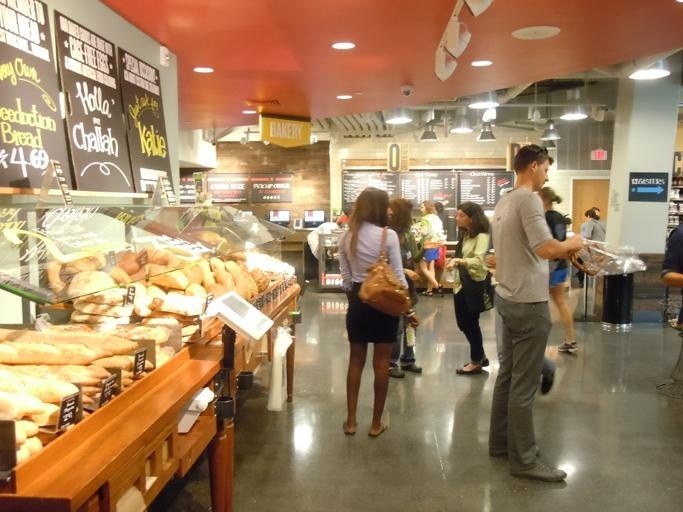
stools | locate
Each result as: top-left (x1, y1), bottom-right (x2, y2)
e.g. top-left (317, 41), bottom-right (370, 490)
top-left (661, 271), bottom-right (683, 328)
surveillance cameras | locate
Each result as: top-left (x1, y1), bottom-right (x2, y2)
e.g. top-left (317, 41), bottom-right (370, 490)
top-left (400, 85), bottom-right (412, 96)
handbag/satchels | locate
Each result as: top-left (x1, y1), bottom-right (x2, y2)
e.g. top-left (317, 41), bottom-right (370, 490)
top-left (461, 280), bottom-right (493, 312)
top-left (359, 257), bottom-right (410, 316)
top-left (434, 246), bottom-right (446, 268)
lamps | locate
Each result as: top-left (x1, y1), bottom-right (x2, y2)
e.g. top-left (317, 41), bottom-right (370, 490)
top-left (629, 56), bottom-right (671, 81)
top-left (380, 108), bottom-right (415, 125)
top-left (476, 122), bottom-right (494, 141)
top-left (541, 121), bottom-right (560, 140)
top-left (540, 142), bottom-right (558, 150)
top-left (467, 90), bottom-right (501, 112)
top-left (555, 87), bottom-right (590, 122)
top-left (239, 127), bottom-right (319, 148)
top-left (419, 121), bottom-right (438, 142)
top-left (448, 108), bottom-right (474, 137)
top-left (431, 0), bottom-right (496, 87)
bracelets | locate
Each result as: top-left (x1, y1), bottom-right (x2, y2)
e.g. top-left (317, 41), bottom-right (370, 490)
top-left (401, 308), bottom-right (416, 317)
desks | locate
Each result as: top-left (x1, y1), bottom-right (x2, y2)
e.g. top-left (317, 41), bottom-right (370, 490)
top-left (143, 274), bottom-right (301, 478)
top-left (0, 346), bottom-right (236, 512)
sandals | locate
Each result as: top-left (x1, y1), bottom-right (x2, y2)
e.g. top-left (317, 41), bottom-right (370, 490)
top-left (437, 284), bottom-right (444, 297)
top-left (421, 291), bottom-right (433, 296)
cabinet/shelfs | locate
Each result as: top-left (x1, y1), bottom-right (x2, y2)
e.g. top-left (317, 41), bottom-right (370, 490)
top-left (666, 176), bottom-right (682, 240)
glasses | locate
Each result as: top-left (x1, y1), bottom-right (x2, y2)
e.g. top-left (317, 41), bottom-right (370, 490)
top-left (531, 147), bottom-right (549, 155)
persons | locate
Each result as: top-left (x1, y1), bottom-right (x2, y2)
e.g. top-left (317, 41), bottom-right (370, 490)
top-left (415, 201), bottom-right (448, 294)
top-left (304, 222), bottom-right (343, 280)
top-left (660, 189), bottom-right (683, 341)
top-left (487, 142), bottom-right (588, 484)
top-left (537, 186), bottom-right (581, 357)
top-left (385, 198), bottom-right (444, 378)
top-left (337, 205), bottom-right (352, 226)
top-left (444, 199), bottom-right (497, 376)
top-left (581, 207), bottom-right (606, 241)
top-left (336, 186), bottom-right (419, 439)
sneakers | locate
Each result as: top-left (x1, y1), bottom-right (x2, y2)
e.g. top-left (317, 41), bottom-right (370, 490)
top-left (513, 459), bottom-right (567, 482)
top-left (558, 340), bottom-right (579, 353)
top-left (402, 363), bottom-right (423, 373)
top-left (541, 374), bottom-right (554, 394)
top-left (389, 363), bottom-right (405, 378)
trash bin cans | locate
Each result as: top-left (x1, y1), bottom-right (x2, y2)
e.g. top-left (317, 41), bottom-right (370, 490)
top-left (602, 272), bottom-right (634, 332)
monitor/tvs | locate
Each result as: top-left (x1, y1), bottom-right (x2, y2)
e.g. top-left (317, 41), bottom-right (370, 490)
top-left (213, 290), bottom-right (274, 342)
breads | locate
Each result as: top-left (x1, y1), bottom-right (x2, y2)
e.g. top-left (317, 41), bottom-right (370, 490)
top-left (568, 242), bottom-right (608, 276)
top-left (0, 231), bottom-right (283, 467)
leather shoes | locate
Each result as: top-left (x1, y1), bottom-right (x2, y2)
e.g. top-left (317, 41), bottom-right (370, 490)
top-left (456, 358), bottom-right (489, 373)
